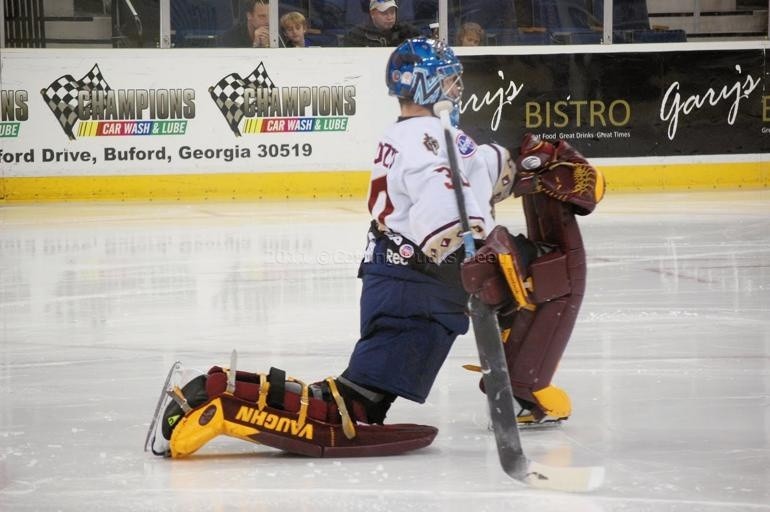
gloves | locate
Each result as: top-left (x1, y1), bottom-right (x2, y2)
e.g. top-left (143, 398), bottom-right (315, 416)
top-left (460, 225), bottom-right (537, 310)
top-left (514, 134), bottom-right (606, 216)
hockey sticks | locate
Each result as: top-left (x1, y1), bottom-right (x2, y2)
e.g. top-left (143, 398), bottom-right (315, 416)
top-left (433, 98), bottom-right (608, 493)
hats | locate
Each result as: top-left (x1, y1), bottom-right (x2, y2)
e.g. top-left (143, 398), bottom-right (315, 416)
top-left (368, 0), bottom-right (398, 13)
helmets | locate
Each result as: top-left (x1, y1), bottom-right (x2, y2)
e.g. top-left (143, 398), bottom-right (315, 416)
top-left (385, 36), bottom-right (464, 106)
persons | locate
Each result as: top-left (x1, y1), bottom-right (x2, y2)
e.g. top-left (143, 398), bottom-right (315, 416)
top-left (455, 22), bottom-right (486, 46)
top-left (342, 0), bottom-right (416, 47)
top-left (211, 1), bottom-right (269, 48)
top-left (281, 12), bottom-right (318, 47)
top-left (158, 36), bottom-right (606, 461)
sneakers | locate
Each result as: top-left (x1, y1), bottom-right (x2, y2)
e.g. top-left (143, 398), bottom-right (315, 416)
top-left (478, 377), bottom-right (569, 422)
top-left (151, 367), bottom-right (207, 456)
